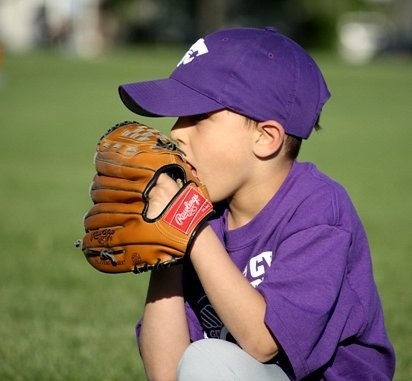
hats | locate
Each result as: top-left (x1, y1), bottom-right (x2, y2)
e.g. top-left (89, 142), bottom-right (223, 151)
top-left (116, 26), bottom-right (330, 139)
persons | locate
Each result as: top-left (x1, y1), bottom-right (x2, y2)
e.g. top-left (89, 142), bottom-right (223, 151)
top-left (118, 26), bottom-right (395, 381)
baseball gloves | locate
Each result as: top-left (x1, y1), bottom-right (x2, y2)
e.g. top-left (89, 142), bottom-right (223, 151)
top-left (74, 120), bottom-right (215, 273)
